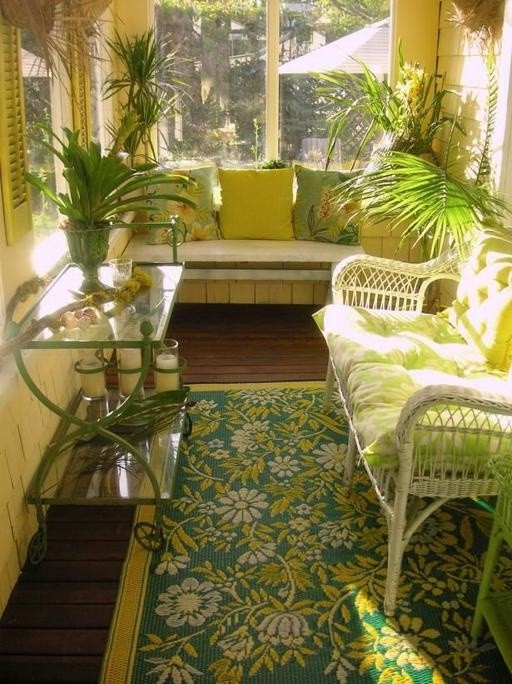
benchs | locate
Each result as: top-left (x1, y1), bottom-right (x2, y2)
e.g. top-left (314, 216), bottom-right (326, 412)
top-left (103, 198), bottom-right (366, 307)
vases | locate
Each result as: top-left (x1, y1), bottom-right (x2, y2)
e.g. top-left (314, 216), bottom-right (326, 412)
top-left (418, 153), bottom-right (435, 165)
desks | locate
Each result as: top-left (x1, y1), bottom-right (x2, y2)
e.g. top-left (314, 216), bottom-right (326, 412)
top-left (11, 261), bottom-right (194, 569)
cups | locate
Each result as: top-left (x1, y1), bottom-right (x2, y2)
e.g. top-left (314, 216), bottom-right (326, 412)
top-left (108, 258), bottom-right (133, 287)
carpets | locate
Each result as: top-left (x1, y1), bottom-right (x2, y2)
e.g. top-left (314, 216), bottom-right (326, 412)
top-left (100, 374), bottom-right (512, 683)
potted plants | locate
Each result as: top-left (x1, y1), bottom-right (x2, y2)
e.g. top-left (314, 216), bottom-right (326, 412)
top-left (97, 25), bottom-right (202, 204)
top-left (21, 120), bottom-right (203, 273)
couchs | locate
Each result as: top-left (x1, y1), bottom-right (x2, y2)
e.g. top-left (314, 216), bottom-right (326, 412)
top-left (317, 237), bottom-right (511, 621)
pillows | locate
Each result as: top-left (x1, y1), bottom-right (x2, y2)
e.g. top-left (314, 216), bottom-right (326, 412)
top-left (139, 166), bottom-right (215, 247)
top-left (217, 165), bottom-right (297, 243)
top-left (291, 165), bottom-right (362, 246)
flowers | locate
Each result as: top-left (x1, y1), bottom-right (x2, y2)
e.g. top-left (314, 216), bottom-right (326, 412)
top-left (307, 51), bottom-right (467, 159)
